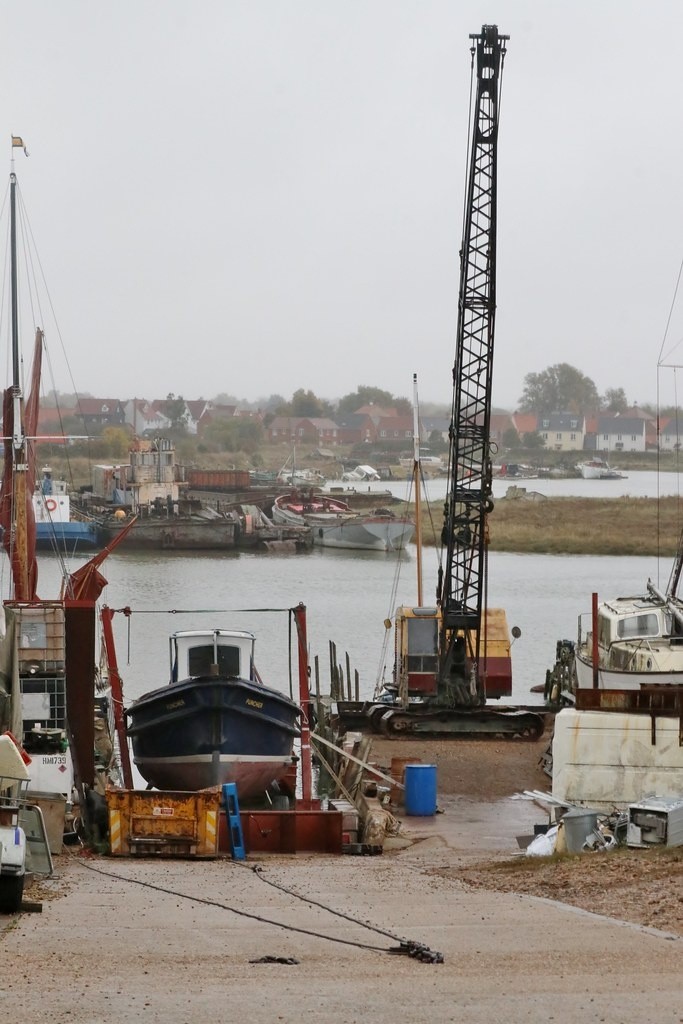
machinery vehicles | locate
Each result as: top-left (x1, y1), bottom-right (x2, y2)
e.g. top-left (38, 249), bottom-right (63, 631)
top-left (366, 22), bottom-right (549, 741)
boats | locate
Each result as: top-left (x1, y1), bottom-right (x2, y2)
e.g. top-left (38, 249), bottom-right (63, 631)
top-left (187, 468), bottom-right (251, 491)
top-left (65, 437), bottom-right (236, 549)
top-left (30, 465), bottom-right (101, 551)
top-left (381, 372), bottom-right (515, 701)
top-left (577, 463), bottom-right (611, 479)
top-left (559, 578), bottom-right (683, 710)
top-left (124, 628), bottom-right (302, 806)
top-left (341, 464), bottom-right (378, 484)
top-left (271, 489), bottom-right (415, 550)
top-left (215, 505), bottom-right (313, 549)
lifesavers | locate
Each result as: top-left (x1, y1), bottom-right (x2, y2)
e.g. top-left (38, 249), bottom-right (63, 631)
top-left (45, 500), bottom-right (56, 510)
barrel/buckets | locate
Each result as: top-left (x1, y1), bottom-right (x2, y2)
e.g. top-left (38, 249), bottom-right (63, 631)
top-left (390, 757), bottom-right (422, 806)
top-left (405, 764), bottom-right (437, 816)
top-left (561, 806), bottom-right (598, 855)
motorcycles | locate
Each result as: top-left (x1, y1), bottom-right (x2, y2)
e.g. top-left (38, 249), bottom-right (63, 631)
top-left (0, 772), bottom-right (32, 912)
